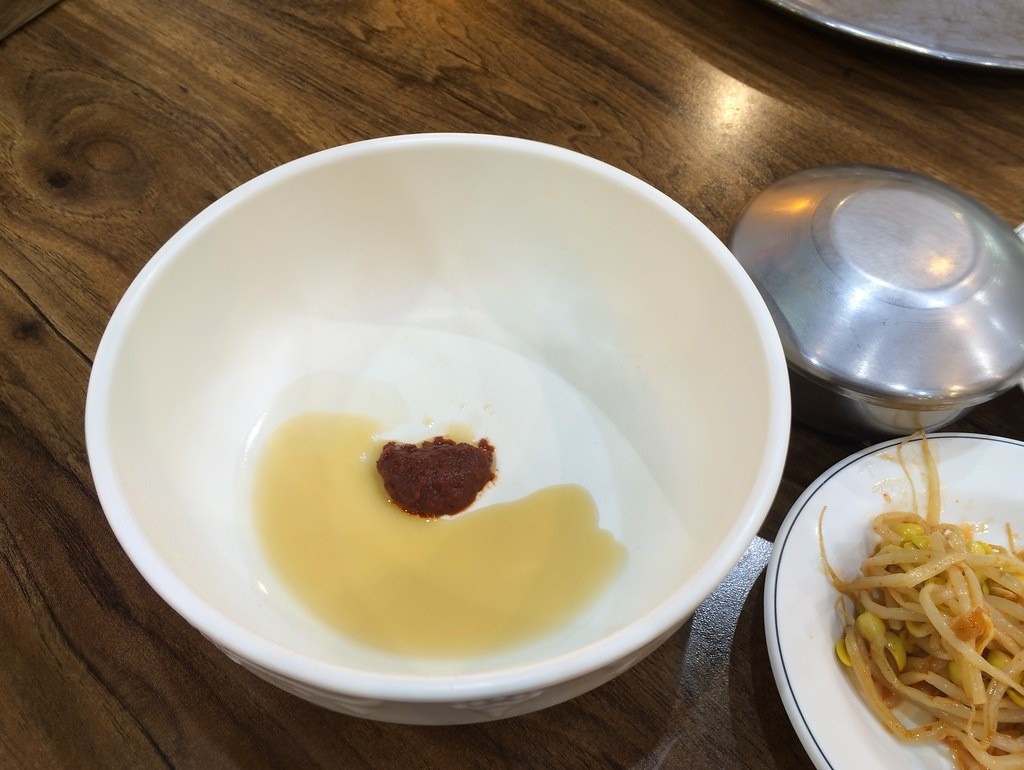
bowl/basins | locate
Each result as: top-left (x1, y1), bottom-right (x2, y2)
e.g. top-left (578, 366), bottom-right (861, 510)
top-left (85, 129), bottom-right (790, 728)
top-left (790, 374), bottom-right (979, 436)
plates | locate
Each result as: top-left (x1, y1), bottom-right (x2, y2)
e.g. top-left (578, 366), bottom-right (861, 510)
top-left (764, 430), bottom-right (1023, 770)
top-left (770, 0), bottom-right (1024, 72)
